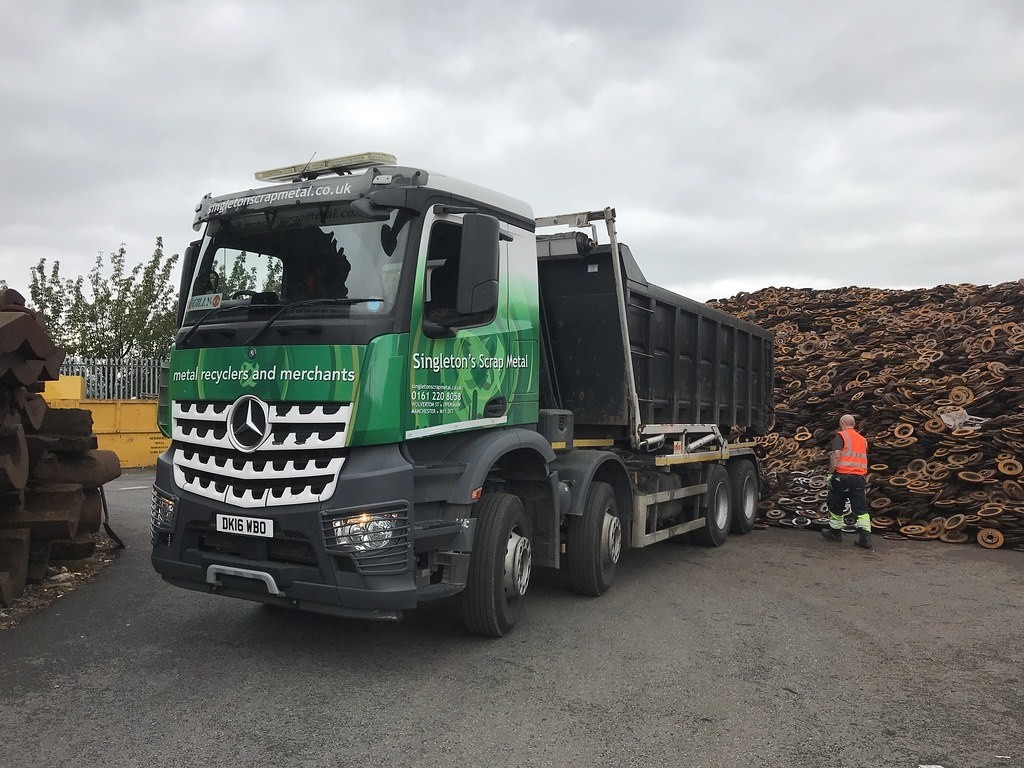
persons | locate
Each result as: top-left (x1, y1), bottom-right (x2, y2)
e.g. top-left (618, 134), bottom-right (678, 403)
top-left (820, 414), bottom-right (873, 549)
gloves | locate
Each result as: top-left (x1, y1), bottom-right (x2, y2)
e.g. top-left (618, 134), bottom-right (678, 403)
top-left (825, 473), bottom-right (833, 490)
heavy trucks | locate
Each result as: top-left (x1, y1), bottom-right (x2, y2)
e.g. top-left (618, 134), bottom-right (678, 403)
top-left (150, 151), bottom-right (777, 638)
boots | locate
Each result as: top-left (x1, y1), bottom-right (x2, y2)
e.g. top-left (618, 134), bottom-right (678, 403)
top-left (821, 528), bottom-right (843, 543)
top-left (853, 531), bottom-right (873, 549)
